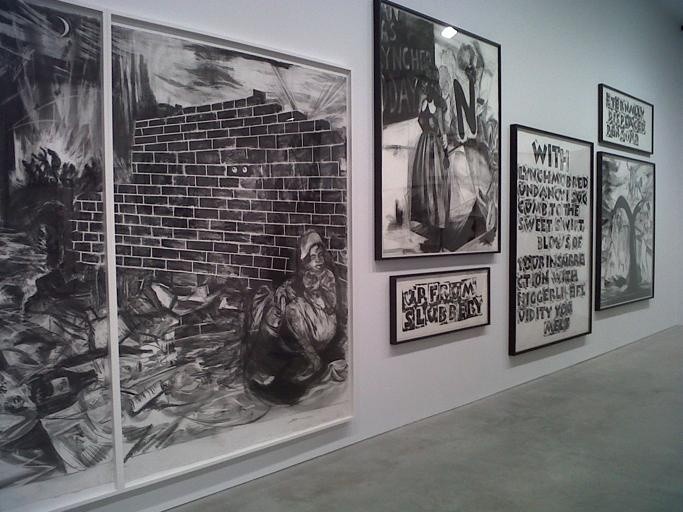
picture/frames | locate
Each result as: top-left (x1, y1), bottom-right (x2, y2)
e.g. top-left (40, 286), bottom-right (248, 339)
top-left (508, 123), bottom-right (594, 355)
top-left (373, 0), bottom-right (501, 261)
top-left (595, 151), bottom-right (655, 310)
top-left (598, 83), bottom-right (654, 154)
top-left (389, 267), bottom-right (491, 345)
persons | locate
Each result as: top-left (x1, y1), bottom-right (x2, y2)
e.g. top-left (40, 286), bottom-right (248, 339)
top-left (410, 64), bottom-right (450, 252)
top-left (253, 228), bottom-right (338, 386)
